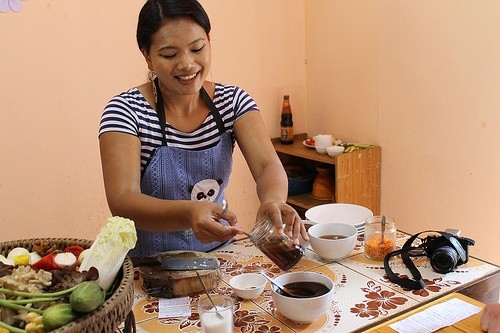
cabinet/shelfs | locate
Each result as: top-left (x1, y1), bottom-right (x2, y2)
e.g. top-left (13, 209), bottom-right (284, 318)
top-left (269, 132), bottom-right (381, 219)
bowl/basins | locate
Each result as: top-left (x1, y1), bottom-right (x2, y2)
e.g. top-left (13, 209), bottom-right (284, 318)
top-left (326, 146), bottom-right (345, 158)
top-left (307, 222), bottom-right (358, 261)
top-left (270, 271), bottom-right (335, 324)
top-left (229, 272), bottom-right (267, 300)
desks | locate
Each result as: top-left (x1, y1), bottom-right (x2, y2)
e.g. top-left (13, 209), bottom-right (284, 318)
top-left (361, 292), bottom-right (487, 333)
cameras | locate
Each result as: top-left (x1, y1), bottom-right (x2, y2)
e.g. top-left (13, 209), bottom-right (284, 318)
top-left (426, 228), bottom-right (475, 274)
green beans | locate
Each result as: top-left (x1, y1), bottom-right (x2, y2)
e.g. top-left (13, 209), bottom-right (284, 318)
top-left (0, 283), bottom-right (89, 333)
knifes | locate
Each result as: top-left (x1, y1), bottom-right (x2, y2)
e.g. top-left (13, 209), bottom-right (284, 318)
top-left (132, 257), bottom-right (218, 270)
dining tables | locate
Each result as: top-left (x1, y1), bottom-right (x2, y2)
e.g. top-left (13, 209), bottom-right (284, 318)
top-left (117, 221), bottom-right (500, 333)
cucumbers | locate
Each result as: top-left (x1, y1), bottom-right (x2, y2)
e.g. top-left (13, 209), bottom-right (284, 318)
top-left (70, 283), bottom-right (105, 313)
top-left (41, 303), bottom-right (73, 329)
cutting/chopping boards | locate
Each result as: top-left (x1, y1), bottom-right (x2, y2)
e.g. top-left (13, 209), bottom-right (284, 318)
top-left (138, 250), bottom-right (222, 299)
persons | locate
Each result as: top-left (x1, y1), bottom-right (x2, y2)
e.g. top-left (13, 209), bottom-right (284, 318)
top-left (98, 0), bottom-right (310, 267)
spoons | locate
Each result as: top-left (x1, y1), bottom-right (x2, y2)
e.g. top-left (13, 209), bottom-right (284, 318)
top-left (196, 271), bottom-right (224, 320)
top-left (260, 271), bottom-right (308, 298)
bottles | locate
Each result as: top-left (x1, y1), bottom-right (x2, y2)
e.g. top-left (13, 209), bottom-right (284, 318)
top-left (280, 95), bottom-right (294, 145)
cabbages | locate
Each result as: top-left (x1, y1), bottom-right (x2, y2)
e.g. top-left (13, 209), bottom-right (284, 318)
top-left (78, 215), bottom-right (138, 290)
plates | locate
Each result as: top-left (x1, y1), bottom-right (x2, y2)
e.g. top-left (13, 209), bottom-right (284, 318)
top-left (304, 203), bottom-right (373, 238)
top-left (303, 139), bottom-right (342, 148)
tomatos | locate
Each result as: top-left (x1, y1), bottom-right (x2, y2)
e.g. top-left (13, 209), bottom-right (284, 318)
top-left (31, 253), bottom-right (54, 272)
top-left (65, 246), bottom-right (84, 259)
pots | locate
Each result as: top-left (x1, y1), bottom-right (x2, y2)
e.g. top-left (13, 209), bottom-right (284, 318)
top-left (284, 166), bottom-right (314, 195)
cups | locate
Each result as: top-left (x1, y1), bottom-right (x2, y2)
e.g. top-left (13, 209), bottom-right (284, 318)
top-left (197, 295), bottom-right (233, 333)
top-left (248, 216), bottom-right (305, 272)
top-left (315, 135), bottom-right (332, 154)
top-left (364, 216), bottom-right (396, 261)
